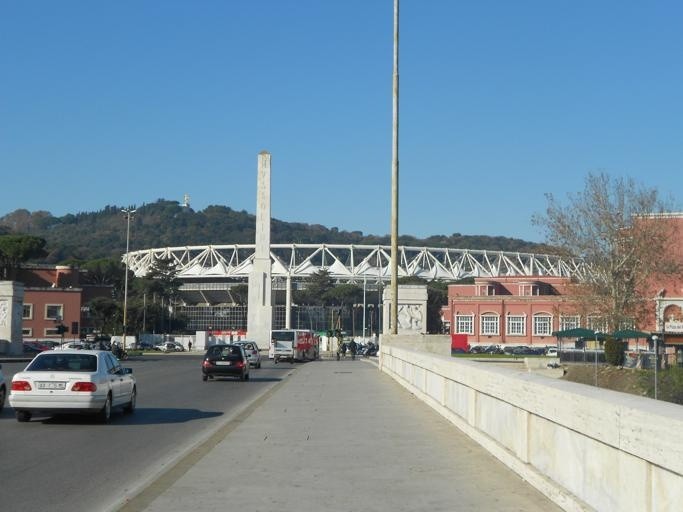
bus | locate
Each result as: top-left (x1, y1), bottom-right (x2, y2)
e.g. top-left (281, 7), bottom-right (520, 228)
top-left (269, 330), bottom-right (320, 364)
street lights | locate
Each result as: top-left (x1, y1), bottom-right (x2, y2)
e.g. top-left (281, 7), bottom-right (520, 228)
top-left (121, 208), bottom-right (136, 354)
top-left (652, 336), bottom-right (659, 398)
top-left (595, 330), bottom-right (598, 385)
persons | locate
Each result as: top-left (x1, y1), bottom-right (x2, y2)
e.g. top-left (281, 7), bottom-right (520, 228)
top-left (366, 342), bottom-right (375, 357)
top-left (116, 341), bottom-right (123, 360)
top-left (187, 340), bottom-right (192, 352)
top-left (347, 338), bottom-right (357, 361)
top-left (111, 340), bottom-right (119, 360)
top-left (341, 343), bottom-right (346, 358)
top-left (336, 343), bottom-right (341, 361)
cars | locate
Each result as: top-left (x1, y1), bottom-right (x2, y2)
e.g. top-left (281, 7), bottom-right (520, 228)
top-left (130, 340), bottom-right (184, 353)
top-left (25, 340), bottom-right (97, 351)
top-left (232, 341), bottom-right (262, 370)
top-left (202, 344), bottom-right (251, 381)
top-left (8, 350), bottom-right (137, 422)
